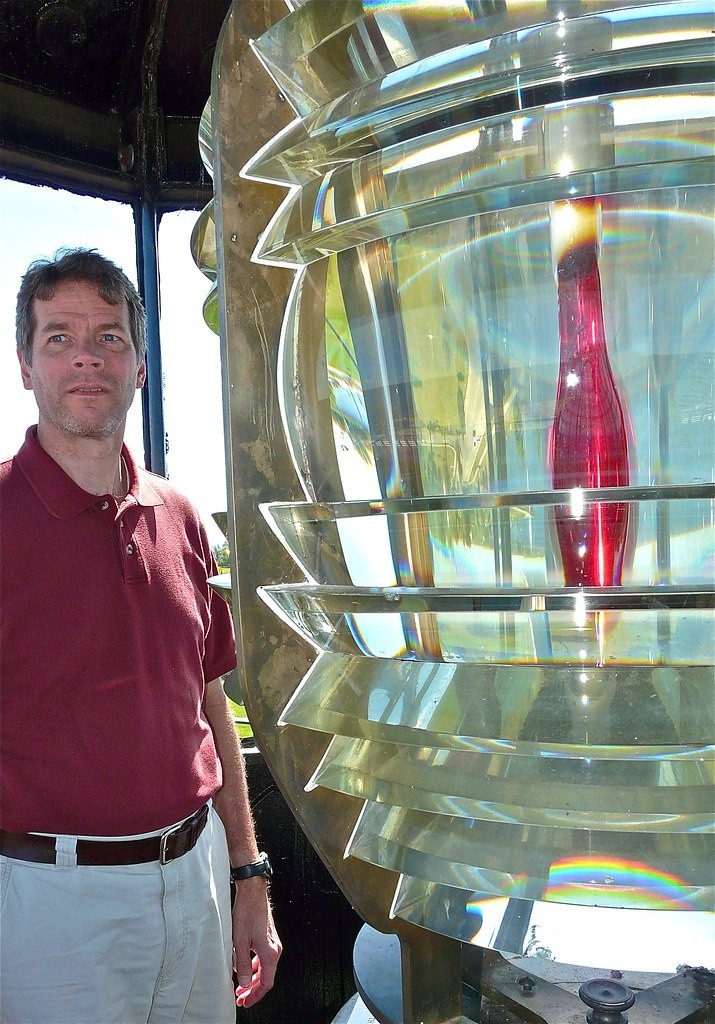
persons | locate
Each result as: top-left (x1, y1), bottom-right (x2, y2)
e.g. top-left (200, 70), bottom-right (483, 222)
top-left (0, 249), bottom-right (285, 1024)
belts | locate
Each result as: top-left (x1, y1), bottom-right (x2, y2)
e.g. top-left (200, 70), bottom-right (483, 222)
top-left (0, 804), bottom-right (209, 866)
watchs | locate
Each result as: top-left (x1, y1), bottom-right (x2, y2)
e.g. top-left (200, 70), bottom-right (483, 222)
top-left (228, 851), bottom-right (274, 881)
